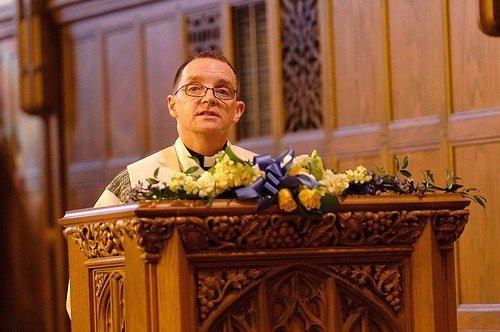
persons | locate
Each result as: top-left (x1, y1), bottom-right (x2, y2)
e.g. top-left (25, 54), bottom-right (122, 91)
top-left (66, 47), bottom-right (276, 320)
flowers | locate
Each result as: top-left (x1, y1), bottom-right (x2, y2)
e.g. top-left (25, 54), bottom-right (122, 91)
top-left (122, 139), bottom-right (487, 218)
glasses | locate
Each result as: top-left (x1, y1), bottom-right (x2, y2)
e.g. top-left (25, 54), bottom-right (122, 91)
top-left (174, 84), bottom-right (237, 100)
